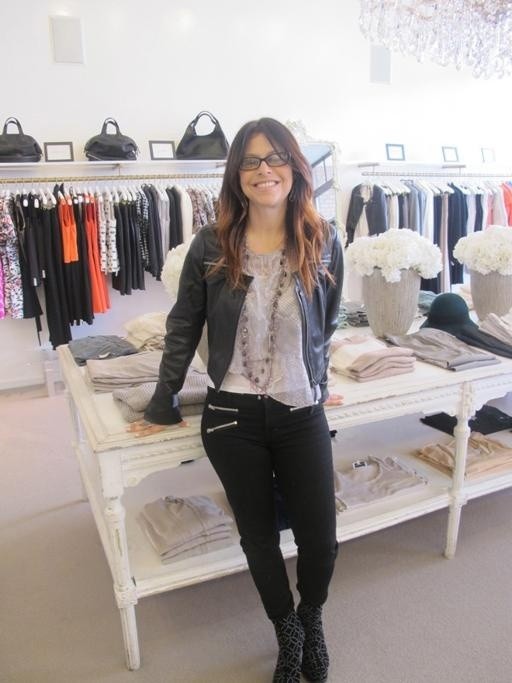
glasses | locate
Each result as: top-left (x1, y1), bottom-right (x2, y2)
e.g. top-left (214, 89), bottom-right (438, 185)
top-left (237, 151), bottom-right (291, 170)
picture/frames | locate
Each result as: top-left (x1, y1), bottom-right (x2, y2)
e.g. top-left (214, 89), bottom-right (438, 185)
top-left (147, 138), bottom-right (176, 159)
top-left (479, 146), bottom-right (498, 163)
top-left (440, 144), bottom-right (459, 162)
top-left (385, 141), bottom-right (406, 160)
top-left (44, 140), bottom-right (74, 162)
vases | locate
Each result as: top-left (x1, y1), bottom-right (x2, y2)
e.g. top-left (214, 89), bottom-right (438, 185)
top-left (195, 307), bottom-right (210, 361)
top-left (361, 262), bottom-right (423, 340)
top-left (465, 264), bottom-right (511, 322)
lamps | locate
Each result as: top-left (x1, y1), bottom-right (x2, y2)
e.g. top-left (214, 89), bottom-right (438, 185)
top-left (355, 0), bottom-right (511, 91)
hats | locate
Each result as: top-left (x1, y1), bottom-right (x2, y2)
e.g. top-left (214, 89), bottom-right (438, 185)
top-left (418, 293), bottom-right (479, 330)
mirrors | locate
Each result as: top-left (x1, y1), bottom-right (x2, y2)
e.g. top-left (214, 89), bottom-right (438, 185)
top-left (280, 119), bottom-right (338, 236)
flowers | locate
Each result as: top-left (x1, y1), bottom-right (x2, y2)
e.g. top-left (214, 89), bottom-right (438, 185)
top-left (451, 223), bottom-right (511, 277)
top-left (338, 223), bottom-right (448, 289)
top-left (160, 229), bottom-right (196, 303)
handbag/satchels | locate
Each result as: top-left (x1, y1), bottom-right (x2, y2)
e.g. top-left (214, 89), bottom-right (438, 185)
top-left (0, 117), bottom-right (42, 163)
top-left (174, 110), bottom-right (230, 160)
top-left (83, 116), bottom-right (138, 161)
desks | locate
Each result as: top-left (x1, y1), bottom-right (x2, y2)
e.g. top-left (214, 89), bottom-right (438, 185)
top-left (54, 284), bottom-right (512, 683)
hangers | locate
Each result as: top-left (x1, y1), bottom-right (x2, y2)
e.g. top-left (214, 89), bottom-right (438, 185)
top-left (2, 174), bottom-right (224, 206)
top-left (352, 170), bottom-right (512, 197)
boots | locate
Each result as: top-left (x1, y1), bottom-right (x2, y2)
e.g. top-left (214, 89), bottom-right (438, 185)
top-left (270, 603), bottom-right (305, 683)
top-left (296, 598), bottom-right (330, 682)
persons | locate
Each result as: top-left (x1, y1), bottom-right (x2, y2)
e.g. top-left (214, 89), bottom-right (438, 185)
top-left (125, 118), bottom-right (345, 682)
